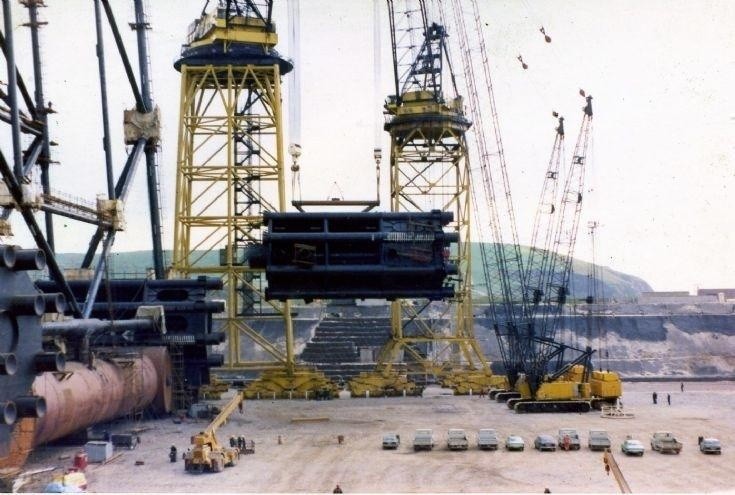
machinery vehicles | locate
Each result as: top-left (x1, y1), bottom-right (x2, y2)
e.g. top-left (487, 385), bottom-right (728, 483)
top-left (182, 390), bottom-right (246, 474)
top-left (436, 0), bottom-right (627, 416)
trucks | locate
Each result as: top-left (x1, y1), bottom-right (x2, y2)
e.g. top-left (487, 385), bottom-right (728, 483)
top-left (447, 429), bottom-right (468, 451)
top-left (589, 428), bottom-right (611, 451)
top-left (558, 427), bottom-right (580, 451)
top-left (650, 430), bottom-right (683, 454)
top-left (412, 429), bottom-right (434, 451)
top-left (477, 428), bottom-right (498, 451)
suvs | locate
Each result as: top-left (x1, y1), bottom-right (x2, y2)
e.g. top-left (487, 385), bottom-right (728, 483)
top-left (700, 437), bottom-right (721, 454)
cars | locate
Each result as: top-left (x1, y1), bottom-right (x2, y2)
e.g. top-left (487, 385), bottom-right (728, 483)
top-left (382, 432), bottom-right (400, 449)
top-left (621, 435), bottom-right (645, 456)
top-left (504, 433), bottom-right (524, 452)
top-left (534, 436), bottom-right (556, 452)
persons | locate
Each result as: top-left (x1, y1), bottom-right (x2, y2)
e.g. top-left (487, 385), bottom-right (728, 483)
top-left (333, 485), bottom-right (343, 494)
top-left (229, 435), bottom-right (246, 450)
top-left (652, 382), bottom-right (704, 445)
top-left (170, 443), bottom-right (177, 452)
